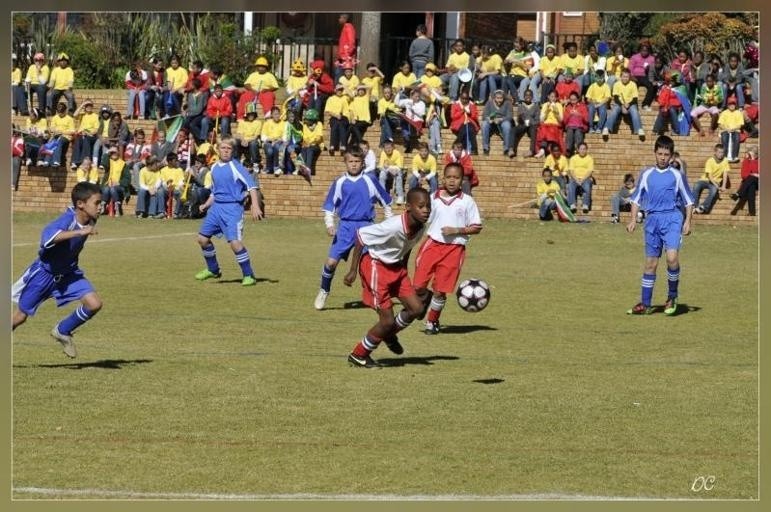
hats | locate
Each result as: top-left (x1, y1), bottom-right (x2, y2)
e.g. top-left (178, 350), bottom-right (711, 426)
top-left (727, 97), bottom-right (737, 105)
top-left (457, 67), bottom-right (472, 83)
top-left (146, 154), bottom-right (162, 163)
top-left (33, 52), bottom-right (44, 61)
top-left (288, 58), bottom-right (307, 71)
top-left (425, 63), bottom-right (436, 72)
top-left (12, 53), bottom-right (18, 60)
top-left (57, 52), bottom-right (70, 61)
top-left (245, 101), bottom-right (256, 114)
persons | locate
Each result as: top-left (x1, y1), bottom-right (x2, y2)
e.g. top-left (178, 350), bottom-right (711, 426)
top-left (534, 165), bottom-right (583, 224)
top-left (568, 144), bottom-right (597, 210)
top-left (24, 51), bottom-right (49, 113)
top-left (324, 13), bottom-right (448, 152)
top-left (213, 55), bottom-right (324, 174)
top-left (413, 162), bottom-right (484, 334)
top-left (611, 171), bottom-right (639, 224)
top-left (356, 141), bottom-right (379, 185)
top-left (542, 144), bottom-right (569, 200)
top-left (11, 123), bottom-right (26, 189)
top-left (11, 182), bottom-right (104, 359)
top-left (72, 54), bottom-right (212, 219)
top-left (668, 152), bottom-right (687, 184)
top-left (437, 142), bottom-right (478, 198)
top-left (314, 145), bottom-right (395, 312)
top-left (11, 52), bottom-right (27, 111)
top-left (50, 51), bottom-right (77, 116)
top-left (375, 140), bottom-right (406, 206)
top-left (687, 142), bottom-right (731, 213)
top-left (448, 37), bottom-right (759, 157)
top-left (43, 101), bottom-right (72, 166)
top-left (410, 142), bottom-right (438, 196)
top-left (25, 129), bottom-right (50, 168)
top-left (343, 186), bottom-right (432, 367)
top-left (22, 108), bottom-right (47, 167)
top-left (627, 134), bottom-right (697, 315)
top-left (194, 134), bottom-right (265, 287)
top-left (243, 160), bottom-right (263, 220)
top-left (734, 142), bottom-right (760, 216)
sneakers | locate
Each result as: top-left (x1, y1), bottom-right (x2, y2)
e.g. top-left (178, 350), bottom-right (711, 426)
top-left (424, 318), bottom-right (440, 335)
top-left (195, 268), bottom-right (222, 280)
top-left (384, 333), bottom-right (403, 354)
top-left (696, 205), bottom-right (704, 214)
top-left (437, 144), bottom-right (444, 154)
top-left (44, 160), bottom-right (49, 167)
top-left (36, 160), bottom-right (44, 168)
top-left (638, 128), bottom-right (645, 137)
top-left (396, 196), bottom-right (405, 205)
top-left (626, 302), bottom-right (652, 314)
top-left (242, 275), bottom-right (256, 286)
top-left (274, 168), bottom-right (284, 176)
top-left (262, 169), bottom-right (273, 174)
top-left (664, 295), bottom-right (678, 314)
top-left (347, 353), bottom-right (383, 369)
top-left (314, 288), bottom-right (329, 310)
top-left (611, 214), bottom-right (619, 224)
top-left (508, 147), bottom-right (515, 158)
top-left (534, 148), bottom-right (545, 160)
top-left (51, 162), bottom-right (61, 167)
top-left (729, 193), bottom-right (738, 200)
top-left (70, 163), bottom-right (77, 168)
top-left (602, 127), bottom-right (609, 137)
top-left (252, 162), bottom-right (261, 173)
top-left (415, 288), bottom-right (433, 320)
top-left (581, 204), bottom-right (590, 211)
top-left (570, 204), bottom-right (578, 211)
top-left (25, 157), bottom-right (33, 166)
top-left (50, 323), bottom-right (77, 358)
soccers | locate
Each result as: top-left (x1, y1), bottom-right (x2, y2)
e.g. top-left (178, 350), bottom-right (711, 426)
top-left (457, 279), bottom-right (490, 312)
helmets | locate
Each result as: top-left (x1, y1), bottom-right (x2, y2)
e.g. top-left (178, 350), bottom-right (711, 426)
top-left (256, 57), bottom-right (268, 66)
top-left (304, 108), bottom-right (320, 120)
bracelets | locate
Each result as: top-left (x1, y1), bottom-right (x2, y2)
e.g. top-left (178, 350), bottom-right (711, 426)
top-left (458, 228), bottom-right (467, 235)
